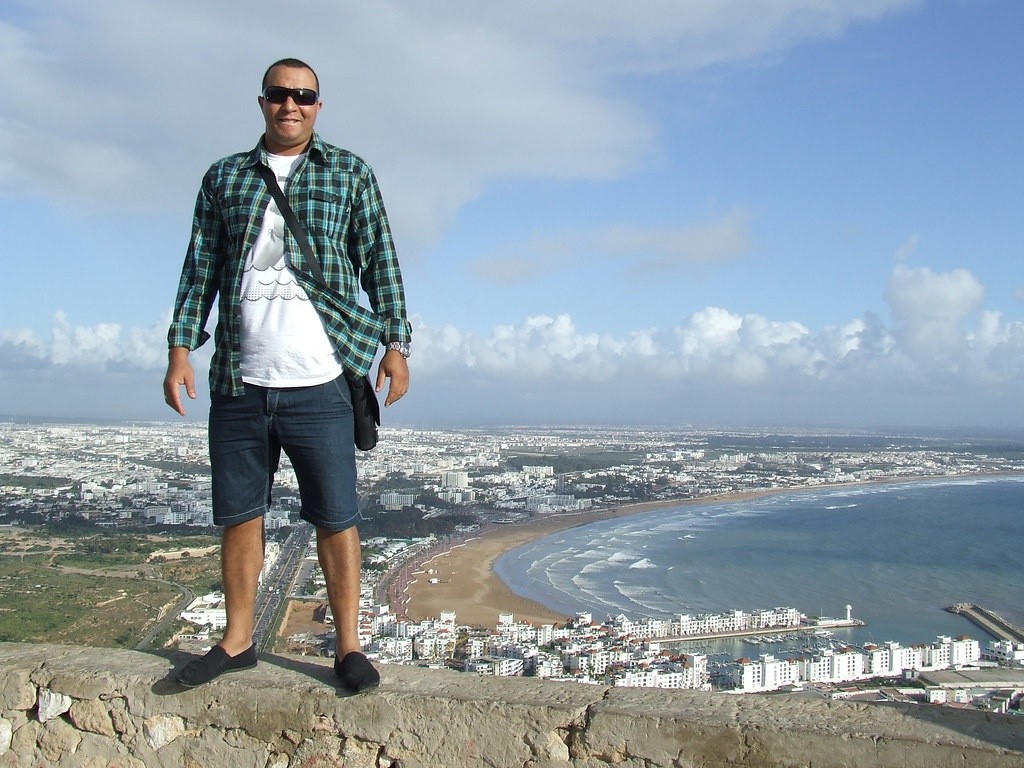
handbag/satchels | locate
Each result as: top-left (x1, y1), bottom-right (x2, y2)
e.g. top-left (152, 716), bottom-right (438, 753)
top-left (351, 372), bottom-right (380, 451)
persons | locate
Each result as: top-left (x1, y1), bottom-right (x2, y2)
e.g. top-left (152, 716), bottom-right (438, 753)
top-left (163, 58), bottom-right (413, 695)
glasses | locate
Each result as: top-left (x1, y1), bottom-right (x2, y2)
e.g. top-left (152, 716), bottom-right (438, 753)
top-left (263, 84), bottom-right (319, 106)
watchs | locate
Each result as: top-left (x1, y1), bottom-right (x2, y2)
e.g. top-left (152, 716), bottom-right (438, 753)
top-left (385, 342), bottom-right (411, 359)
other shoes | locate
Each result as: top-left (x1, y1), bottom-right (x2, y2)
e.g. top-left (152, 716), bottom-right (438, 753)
top-left (334, 651), bottom-right (380, 694)
top-left (176, 642), bottom-right (257, 687)
